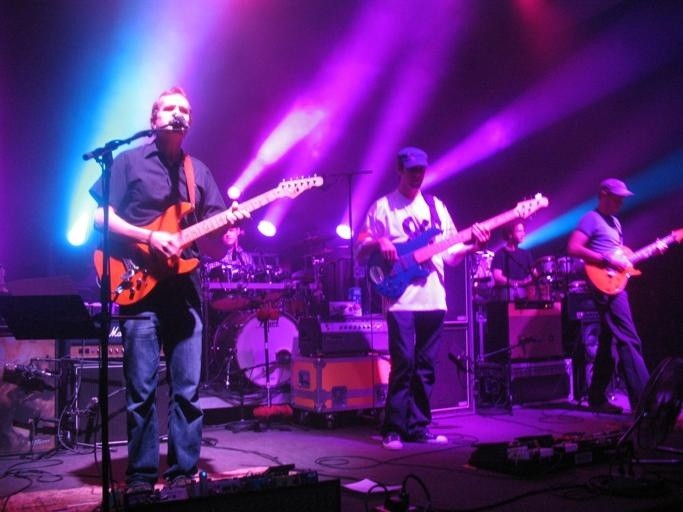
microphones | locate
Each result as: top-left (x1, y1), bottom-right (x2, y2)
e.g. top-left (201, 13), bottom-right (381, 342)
top-left (5, 362), bottom-right (52, 378)
top-left (169, 112), bottom-right (190, 135)
top-left (448, 348), bottom-right (470, 376)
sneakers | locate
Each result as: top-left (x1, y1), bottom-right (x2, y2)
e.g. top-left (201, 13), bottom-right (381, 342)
top-left (592, 400), bottom-right (623, 413)
top-left (416, 432), bottom-right (448, 445)
top-left (383, 434), bottom-right (402, 450)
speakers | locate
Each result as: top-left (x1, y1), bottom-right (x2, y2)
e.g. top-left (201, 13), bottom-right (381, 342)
top-left (0, 294), bottom-right (99, 340)
top-left (479, 357), bottom-right (574, 409)
top-left (496, 302), bottom-right (565, 360)
top-left (564, 311), bottom-right (610, 397)
top-left (74, 362), bottom-right (170, 446)
top-left (0, 334), bottom-right (61, 458)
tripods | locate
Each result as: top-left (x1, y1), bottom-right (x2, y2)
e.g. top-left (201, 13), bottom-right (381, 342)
top-left (204, 312), bottom-right (240, 390)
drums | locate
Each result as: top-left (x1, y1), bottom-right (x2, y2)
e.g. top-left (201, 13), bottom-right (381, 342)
top-left (532, 255), bottom-right (557, 284)
top-left (557, 255), bottom-right (582, 276)
top-left (206, 261), bottom-right (256, 310)
top-left (213, 305), bottom-right (301, 391)
top-left (287, 279), bottom-right (310, 315)
top-left (255, 266), bottom-right (283, 303)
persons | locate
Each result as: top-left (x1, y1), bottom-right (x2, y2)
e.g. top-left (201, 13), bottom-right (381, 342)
top-left (85, 85), bottom-right (251, 493)
top-left (354, 147), bottom-right (493, 452)
top-left (565, 176), bottom-right (670, 412)
top-left (487, 218), bottom-right (542, 287)
top-left (201, 217), bottom-right (256, 312)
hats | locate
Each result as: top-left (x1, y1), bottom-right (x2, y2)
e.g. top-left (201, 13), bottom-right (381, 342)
top-left (600, 179), bottom-right (633, 197)
top-left (399, 148), bottom-right (428, 169)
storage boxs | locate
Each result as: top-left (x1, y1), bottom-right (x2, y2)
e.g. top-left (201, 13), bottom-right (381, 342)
top-left (290, 355), bottom-right (394, 427)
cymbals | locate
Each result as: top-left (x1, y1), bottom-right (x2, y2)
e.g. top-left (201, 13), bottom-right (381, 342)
top-left (276, 234), bottom-right (338, 252)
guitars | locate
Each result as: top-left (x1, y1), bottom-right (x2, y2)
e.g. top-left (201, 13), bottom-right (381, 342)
top-left (367, 194), bottom-right (548, 299)
top-left (92, 173), bottom-right (323, 306)
top-left (584, 229), bottom-right (683, 295)
